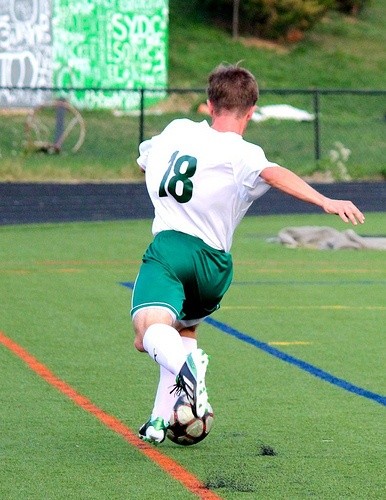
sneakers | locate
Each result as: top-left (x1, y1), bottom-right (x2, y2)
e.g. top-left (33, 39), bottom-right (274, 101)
top-left (138, 417), bottom-right (170, 443)
top-left (172, 347), bottom-right (209, 420)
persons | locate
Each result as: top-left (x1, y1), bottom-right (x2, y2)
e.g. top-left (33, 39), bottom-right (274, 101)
top-left (122, 60), bottom-right (367, 444)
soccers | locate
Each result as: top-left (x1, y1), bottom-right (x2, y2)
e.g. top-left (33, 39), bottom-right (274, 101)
top-left (160, 391), bottom-right (214, 446)
